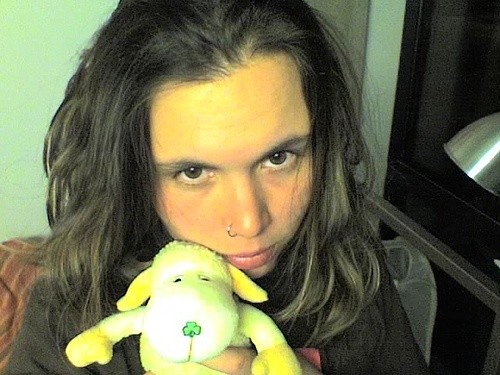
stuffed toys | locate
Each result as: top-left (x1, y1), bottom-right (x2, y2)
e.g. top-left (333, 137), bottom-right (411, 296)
top-left (66, 240), bottom-right (301, 375)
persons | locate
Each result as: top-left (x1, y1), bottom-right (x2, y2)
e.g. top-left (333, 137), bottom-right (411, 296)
top-left (7, 0), bottom-right (431, 375)
